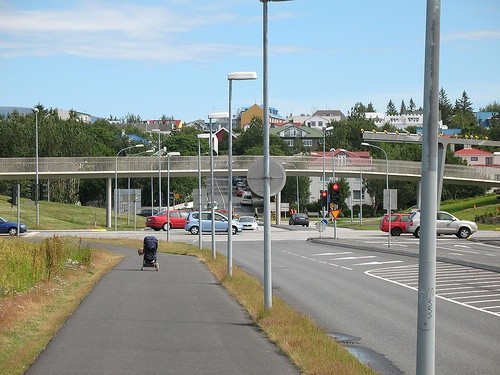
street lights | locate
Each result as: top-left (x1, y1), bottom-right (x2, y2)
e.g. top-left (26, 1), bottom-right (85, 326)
top-left (226, 70), bottom-right (258, 276)
top-left (360, 142), bottom-right (389, 190)
top-left (340, 148), bottom-right (363, 226)
top-left (127, 149), bottom-right (154, 226)
top-left (208, 112), bottom-right (229, 258)
top-left (152, 154), bottom-right (172, 216)
top-left (197, 133), bottom-right (215, 250)
top-left (167, 151), bottom-right (180, 241)
top-left (114, 143), bottom-right (144, 231)
top-left (151, 128), bottom-right (162, 212)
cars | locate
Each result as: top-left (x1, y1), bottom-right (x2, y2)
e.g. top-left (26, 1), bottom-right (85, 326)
top-left (184, 211), bottom-right (242, 235)
top-left (0, 215), bottom-right (28, 236)
top-left (289, 213), bottom-right (309, 227)
top-left (145, 209), bottom-right (190, 231)
top-left (235, 181), bottom-right (246, 190)
top-left (227, 175), bottom-right (243, 186)
top-left (140, 208), bottom-right (158, 217)
top-left (237, 215), bottom-right (259, 231)
top-left (236, 187), bottom-right (244, 196)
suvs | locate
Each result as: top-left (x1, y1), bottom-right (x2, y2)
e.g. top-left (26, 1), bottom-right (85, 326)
top-left (240, 191), bottom-right (254, 206)
top-left (406, 210), bottom-right (478, 239)
top-left (379, 213), bottom-right (413, 237)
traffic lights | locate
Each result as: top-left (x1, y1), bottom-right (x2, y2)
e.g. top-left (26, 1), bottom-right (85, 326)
top-left (6, 183), bottom-right (18, 206)
top-left (329, 182), bottom-right (340, 205)
top-left (26, 182), bottom-right (36, 202)
top-left (319, 189), bottom-right (328, 207)
top-left (39, 182), bottom-right (48, 201)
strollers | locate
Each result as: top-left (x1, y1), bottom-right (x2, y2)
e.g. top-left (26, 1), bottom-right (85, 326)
top-left (137, 236), bottom-right (160, 271)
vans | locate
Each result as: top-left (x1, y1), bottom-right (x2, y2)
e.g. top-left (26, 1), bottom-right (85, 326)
top-left (206, 201), bottom-right (218, 210)
